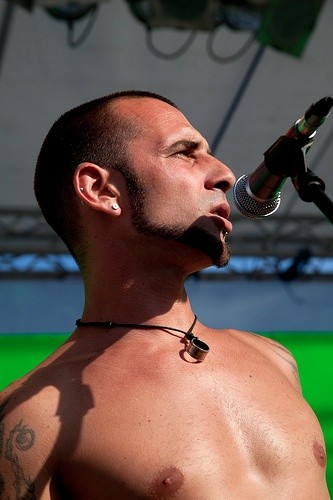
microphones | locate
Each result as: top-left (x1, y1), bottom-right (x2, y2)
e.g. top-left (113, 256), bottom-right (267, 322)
top-left (233, 96), bottom-right (333, 220)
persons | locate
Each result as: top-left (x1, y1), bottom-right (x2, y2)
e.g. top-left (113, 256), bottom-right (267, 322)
top-left (0, 90), bottom-right (333, 500)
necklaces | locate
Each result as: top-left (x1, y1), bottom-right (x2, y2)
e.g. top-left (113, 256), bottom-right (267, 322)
top-left (75, 314), bottom-right (211, 362)
